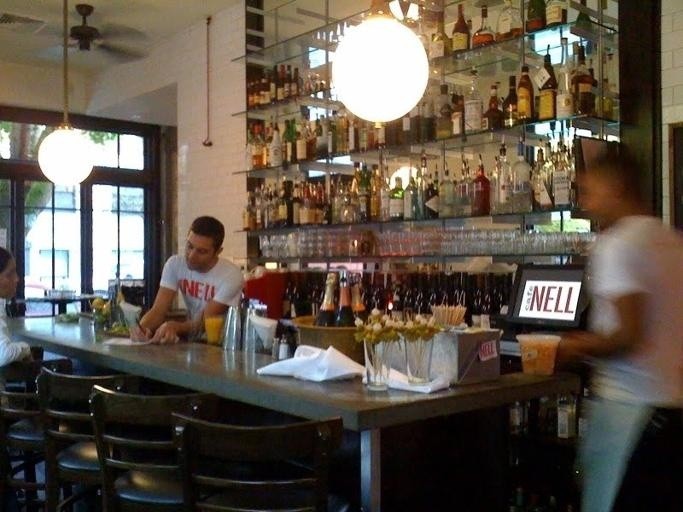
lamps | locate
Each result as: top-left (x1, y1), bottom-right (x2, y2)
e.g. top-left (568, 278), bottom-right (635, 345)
top-left (331, 0), bottom-right (428, 123)
top-left (37, 0), bottom-right (95, 184)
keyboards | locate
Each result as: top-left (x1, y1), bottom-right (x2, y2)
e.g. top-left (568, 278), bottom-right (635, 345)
top-left (499, 339), bottom-right (520, 357)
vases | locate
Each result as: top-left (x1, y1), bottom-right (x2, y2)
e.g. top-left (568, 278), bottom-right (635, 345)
top-left (404, 335), bottom-right (435, 385)
top-left (365, 337), bottom-right (394, 392)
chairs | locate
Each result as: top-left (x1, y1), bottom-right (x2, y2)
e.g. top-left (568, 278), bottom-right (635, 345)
top-left (87, 384), bottom-right (221, 512)
top-left (35, 366), bottom-right (140, 512)
top-left (0, 345), bottom-right (73, 512)
top-left (170, 412), bottom-right (344, 512)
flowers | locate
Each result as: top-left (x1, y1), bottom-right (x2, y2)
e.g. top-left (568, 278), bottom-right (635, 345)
top-left (354, 308), bottom-right (403, 385)
top-left (395, 310), bottom-right (437, 382)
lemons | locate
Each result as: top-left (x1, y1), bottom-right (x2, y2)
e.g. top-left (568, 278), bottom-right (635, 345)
top-left (102, 303), bottom-right (112, 315)
top-left (91, 297), bottom-right (104, 310)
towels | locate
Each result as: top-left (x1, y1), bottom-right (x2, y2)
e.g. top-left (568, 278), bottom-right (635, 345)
top-left (254, 344), bottom-right (364, 383)
top-left (362, 361), bottom-right (450, 394)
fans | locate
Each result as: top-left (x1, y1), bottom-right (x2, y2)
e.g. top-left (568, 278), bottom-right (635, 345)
top-left (17, 4), bottom-right (149, 59)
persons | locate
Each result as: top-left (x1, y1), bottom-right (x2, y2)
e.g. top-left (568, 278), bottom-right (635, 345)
top-left (0, 246), bottom-right (36, 367)
top-left (555, 146), bottom-right (681, 511)
top-left (130, 215), bottom-right (242, 346)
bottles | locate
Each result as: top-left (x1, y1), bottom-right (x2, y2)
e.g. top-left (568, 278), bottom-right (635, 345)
top-left (242, 61), bottom-right (388, 229)
top-left (240, 307), bottom-right (267, 354)
top-left (222, 304), bottom-right (244, 350)
top-left (387, 137), bottom-right (576, 222)
top-left (238, 264), bottom-right (515, 319)
top-left (505, 477), bottom-right (576, 510)
top-left (270, 336), bottom-right (291, 361)
top-left (506, 390), bottom-right (581, 444)
top-left (239, 348), bottom-right (264, 379)
top-left (389, 4), bottom-right (619, 145)
top-left (221, 349), bottom-right (240, 379)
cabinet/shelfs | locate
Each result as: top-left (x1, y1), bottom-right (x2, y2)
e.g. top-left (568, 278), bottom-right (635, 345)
top-left (230, 0), bottom-right (653, 262)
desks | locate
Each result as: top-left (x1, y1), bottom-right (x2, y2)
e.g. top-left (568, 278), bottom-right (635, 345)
top-left (25, 296), bottom-right (103, 316)
top-left (6, 316), bottom-right (583, 511)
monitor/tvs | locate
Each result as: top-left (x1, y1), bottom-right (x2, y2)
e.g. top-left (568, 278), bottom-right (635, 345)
top-left (505, 264), bottom-right (586, 327)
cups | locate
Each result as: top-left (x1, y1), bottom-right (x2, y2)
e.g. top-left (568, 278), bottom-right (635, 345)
top-left (203, 314), bottom-right (225, 344)
top-left (515, 332), bottom-right (562, 376)
top-left (255, 225), bottom-right (598, 266)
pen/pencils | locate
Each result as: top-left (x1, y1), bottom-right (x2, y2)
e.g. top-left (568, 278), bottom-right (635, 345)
top-left (135, 318), bottom-right (146, 335)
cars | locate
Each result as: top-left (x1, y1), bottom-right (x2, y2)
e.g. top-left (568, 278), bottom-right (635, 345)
top-left (24, 285), bottom-right (58, 316)
